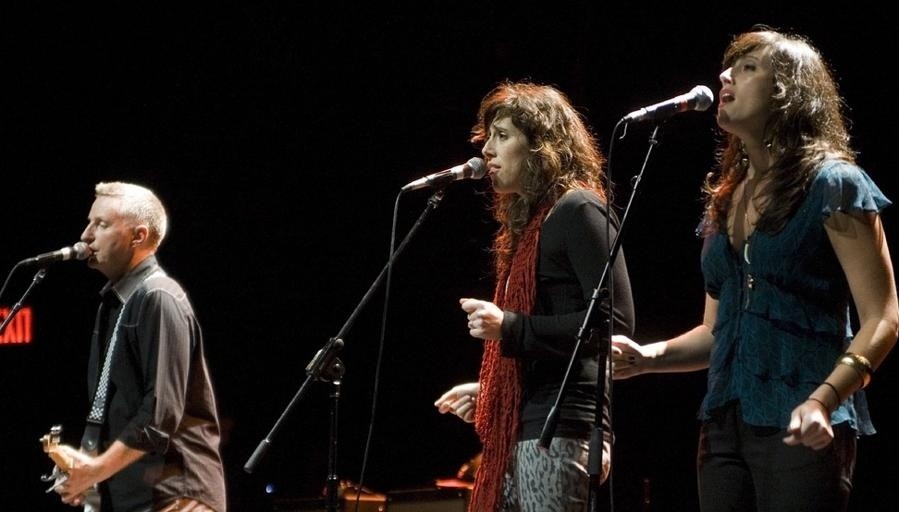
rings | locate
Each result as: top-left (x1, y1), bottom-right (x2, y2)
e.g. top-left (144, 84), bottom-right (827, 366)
top-left (469, 312), bottom-right (473, 319)
top-left (469, 321), bottom-right (475, 328)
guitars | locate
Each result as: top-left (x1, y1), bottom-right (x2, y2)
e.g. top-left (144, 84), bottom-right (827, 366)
top-left (40, 425), bottom-right (101, 512)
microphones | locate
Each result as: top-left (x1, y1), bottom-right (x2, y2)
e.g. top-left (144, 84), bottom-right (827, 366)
top-left (402, 157), bottom-right (485, 194)
top-left (20, 241), bottom-right (91, 267)
top-left (618, 84), bottom-right (716, 123)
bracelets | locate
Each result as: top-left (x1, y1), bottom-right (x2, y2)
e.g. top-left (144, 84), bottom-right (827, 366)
top-left (819, 382), bottom-right (841, 410)
top-left (836, 352), bottom-right (872, 391)
top-left (807, 397), bottom-right (832, 421)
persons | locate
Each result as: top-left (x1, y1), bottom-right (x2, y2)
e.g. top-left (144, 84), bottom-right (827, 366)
top-left (431, 76), bottom-right (636, 511)
top-left (40, 180), bottom-right (229, 511)
top-left (605, 23), bottom-right (899, 511)
